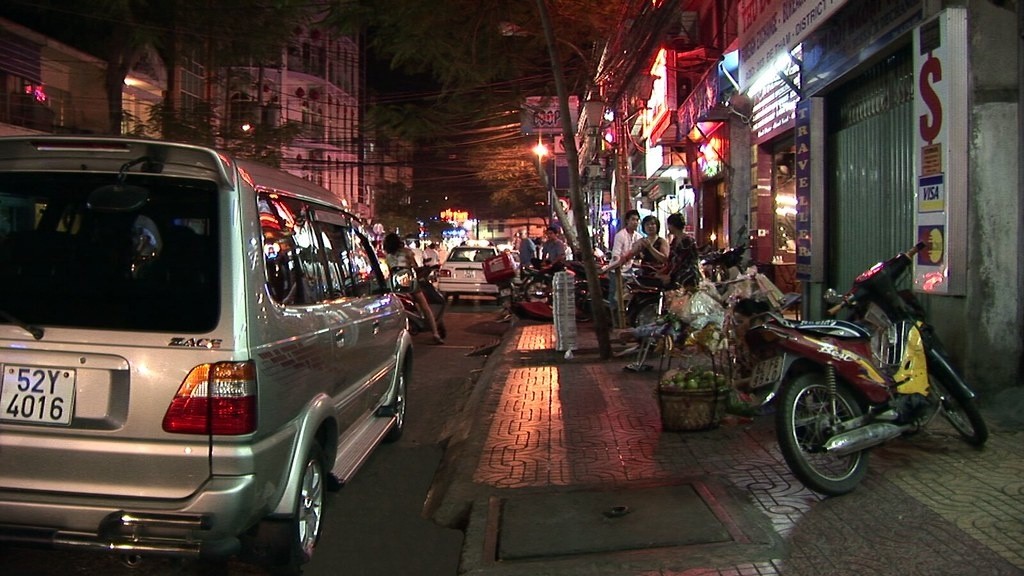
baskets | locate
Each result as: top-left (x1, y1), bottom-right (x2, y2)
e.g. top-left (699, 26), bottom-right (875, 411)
top-left (654, 387), bottom-right (728, 431)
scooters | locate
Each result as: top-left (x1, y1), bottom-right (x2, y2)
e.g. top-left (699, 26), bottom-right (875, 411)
top-left (517, 223), bottom-right (765, 373)
top-left (393, 257), bottom-right (447, 340)
top-left (482, 247), bottom-right (522, 314)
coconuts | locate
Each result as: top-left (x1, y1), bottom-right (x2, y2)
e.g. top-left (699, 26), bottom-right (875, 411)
top-left (661, 369), bottom-right (727, 390)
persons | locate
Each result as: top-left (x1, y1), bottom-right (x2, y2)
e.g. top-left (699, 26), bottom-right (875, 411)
top-left (516, 229), bottom-right (537, 296)
top-left (618, 216), bottom-right (670, 329)
top-left (383, 233), bottom-right (445, 346)
top-left (412, 242), bottom-right (426, 267)
top-left (608, 210), bottom-right (644, 335)
top-left (655, 213), bottom-right (700, 358)
top-left (424, 243), bottom-right (440, 268)
top-left (533, 226), bottom-right (566, 293)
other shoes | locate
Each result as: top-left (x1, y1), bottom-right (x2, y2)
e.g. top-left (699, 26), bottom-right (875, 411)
top-left (427, 338), bottom-right (444, 345)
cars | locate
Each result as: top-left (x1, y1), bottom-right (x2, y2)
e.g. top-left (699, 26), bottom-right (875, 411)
top-left (438, 245), bottom-right (506, 306)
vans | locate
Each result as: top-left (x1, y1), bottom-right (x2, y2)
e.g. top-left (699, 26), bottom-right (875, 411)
top-left (0, 135), bottom-right (418, 564)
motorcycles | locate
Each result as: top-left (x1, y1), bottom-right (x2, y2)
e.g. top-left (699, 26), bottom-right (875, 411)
top-left (745, 241), bottom-right (990, 496)
